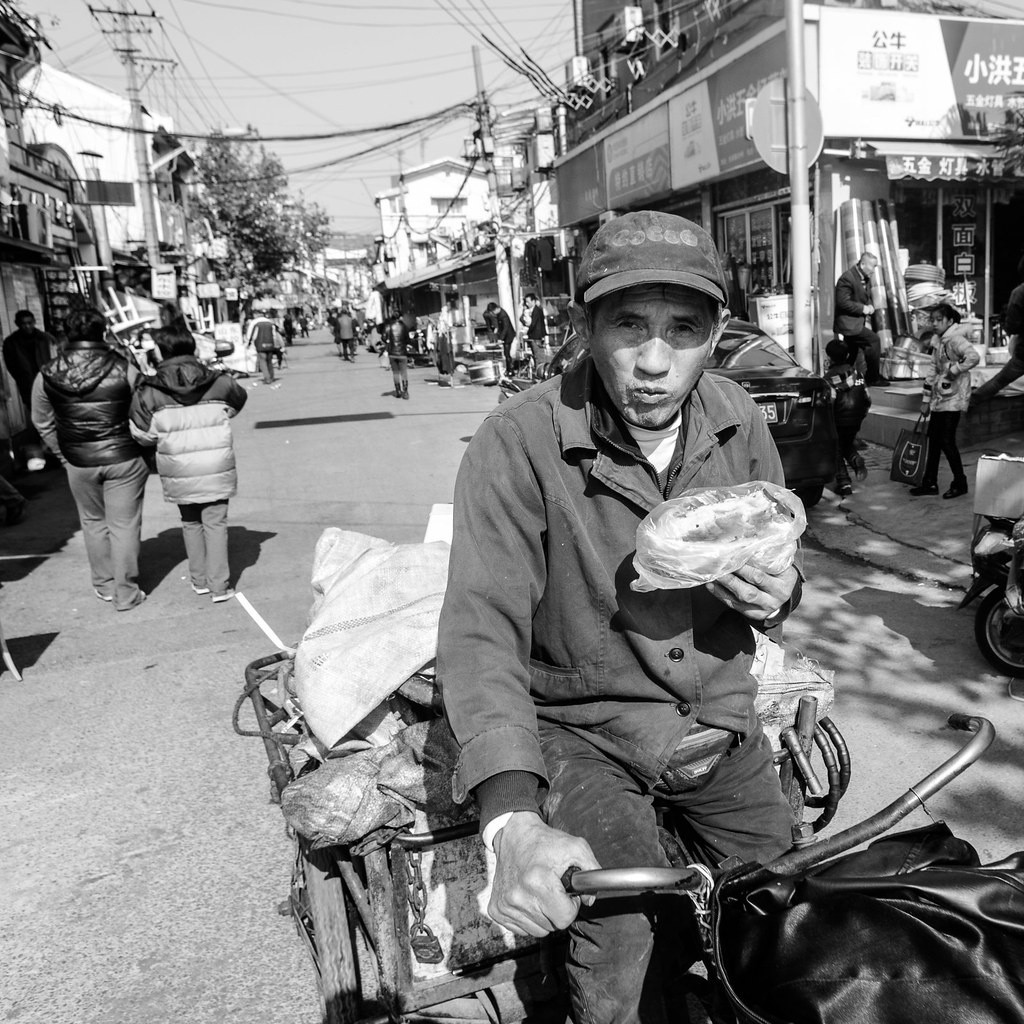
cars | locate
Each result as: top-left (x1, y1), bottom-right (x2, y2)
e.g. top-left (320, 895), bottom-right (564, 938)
top-left (538, 315), bottom-right (837, 509)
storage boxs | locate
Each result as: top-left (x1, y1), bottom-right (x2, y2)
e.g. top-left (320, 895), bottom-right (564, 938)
top-left (879, 346), bottom-right (932, 379)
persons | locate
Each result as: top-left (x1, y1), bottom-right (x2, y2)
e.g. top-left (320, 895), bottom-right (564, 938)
top-left (380, 310), bottom-right (410, 399)
top-left (822, 339), bottom-right (871, 496)
top-left (833, 252), bottom-right (891, 387)
top-left (31, 307), bottom-right (151, 611)
top-left (128, 324), bottom-right (247, 602)
top-left (2, 310), bottom-right (56, 409)
top-left (273, 331), bottom-right (286, 369)
top-left (283, 315), bottom-right (293, 346)
top-left (244, 311), bottom-right (276, 384)
top-left (483, 302), bottom-right (517, 377)
top-left (300, 315), bottom-right (309, 338)
top-left (520, 293), bottom-right (547, 367)
top-left (909, 304), bottom-right (980, 500)
top-left (436, 210), bottom-right (806, 1024)
top-left (336, 309), bottom-right (355, 362)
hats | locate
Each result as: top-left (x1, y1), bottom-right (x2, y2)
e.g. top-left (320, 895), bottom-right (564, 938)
top-left (576, 210), bottom-right (729, 309)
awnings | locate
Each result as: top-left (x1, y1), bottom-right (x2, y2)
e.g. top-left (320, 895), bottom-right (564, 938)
top-left (866, 140), bottom-right (1024, 182)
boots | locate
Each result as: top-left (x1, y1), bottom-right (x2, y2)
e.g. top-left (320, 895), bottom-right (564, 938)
top-left (402, 380), bottom-right (409, 399)
top-left (394, 383), bottom-right (402, 398)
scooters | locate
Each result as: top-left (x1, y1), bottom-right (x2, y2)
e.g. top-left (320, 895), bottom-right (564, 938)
top-left (955, 450), bottom-right (1024, 676)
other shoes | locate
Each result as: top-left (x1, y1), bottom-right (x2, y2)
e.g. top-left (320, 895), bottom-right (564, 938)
top-left (834, 486), bottom-right (852, 495)
top-left (866, 376), bottom-right (891, 388)
top-left (854, 456), bottom-right (868, 482)
top-left (506, 371), bottom-right (515, 376)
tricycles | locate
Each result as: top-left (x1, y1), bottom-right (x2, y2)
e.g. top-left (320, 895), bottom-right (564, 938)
top-left (225, 631), bottom-right (1024, 1024)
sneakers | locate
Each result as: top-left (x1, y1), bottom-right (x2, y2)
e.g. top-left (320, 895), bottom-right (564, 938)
top-left (191, 580), bottom-right (211, 594)
top-left (94, 587), bottom-right (113, 601)
top-left (213, 587), bottom-right (235, 603)
top-left (116, 590), bottom-right (147, 610)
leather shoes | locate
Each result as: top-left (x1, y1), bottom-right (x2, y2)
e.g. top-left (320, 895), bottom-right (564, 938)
top-left (943, 481), bottom-right (969, 498)
top-left (909, 483), bottom-right (940, 496)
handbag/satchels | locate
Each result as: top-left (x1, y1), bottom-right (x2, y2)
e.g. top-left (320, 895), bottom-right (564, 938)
top-left (657, 723), bottom-right (737, 793)
top-left (890, 413), bottom-right (930, 486)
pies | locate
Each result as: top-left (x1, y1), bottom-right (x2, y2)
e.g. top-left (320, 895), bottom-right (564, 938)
top-left (676, 491), bottom-right (788, 542)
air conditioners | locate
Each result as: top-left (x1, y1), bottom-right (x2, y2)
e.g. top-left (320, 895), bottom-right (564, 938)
top-left (554, 232), bottom-right (575, 257)
top-left (7, 202), bottom-right (54, 250)
top-left (599, 211), bottom-right (619, 227)
top-left (614, 7), bottom-right (644, 52)
top-left (530, 106), bottom-right (554, 171)
top-left (565, 56), bottom-right (589, 91)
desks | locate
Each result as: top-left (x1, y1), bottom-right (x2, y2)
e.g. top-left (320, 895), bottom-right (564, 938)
top-left (748, 294), bottom-right (794, 350)
